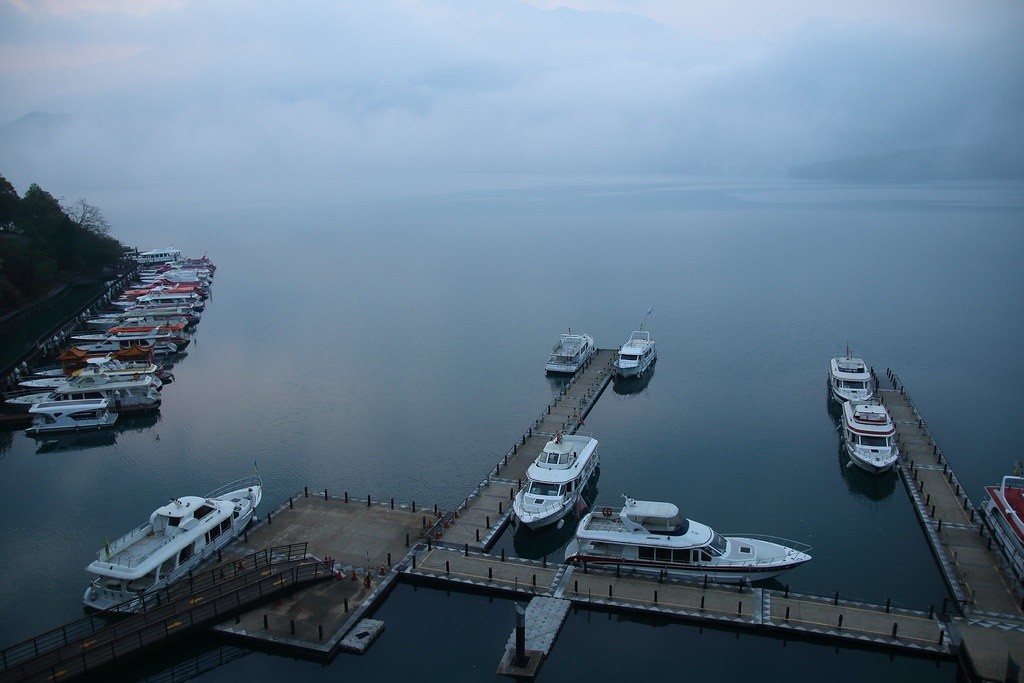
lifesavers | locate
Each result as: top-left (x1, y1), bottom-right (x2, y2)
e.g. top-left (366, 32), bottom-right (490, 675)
top-left (603, 507), bottom-right (612, 517)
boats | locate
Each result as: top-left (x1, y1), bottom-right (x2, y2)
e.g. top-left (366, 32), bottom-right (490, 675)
top-left (563, 492), bottom-right (813, 585)
top-left (79, 460), bottom-right (263, 615)
top-left (67, 244), bottom-right (218, 355)
top-left (513, 428), bottom-right (600, 531)
top-left (610, 322), bottom-right (657, 376)
top-left (24, 397), bottom-right (119, 433)
top-left (5, 369), bottom-right (161, 416)
top-left (547, 325), bottom-right (596, 376)
top-left (19, 361), bottom-right (163, 393)
top-left (980, 473), bottom-right (1024, 586)
top-left (830, 340), bottom-right (876, 408)
top-left (31, 343), bottom-right (174, 380)
top-left (839, 395), bottom-right (898, 475)
